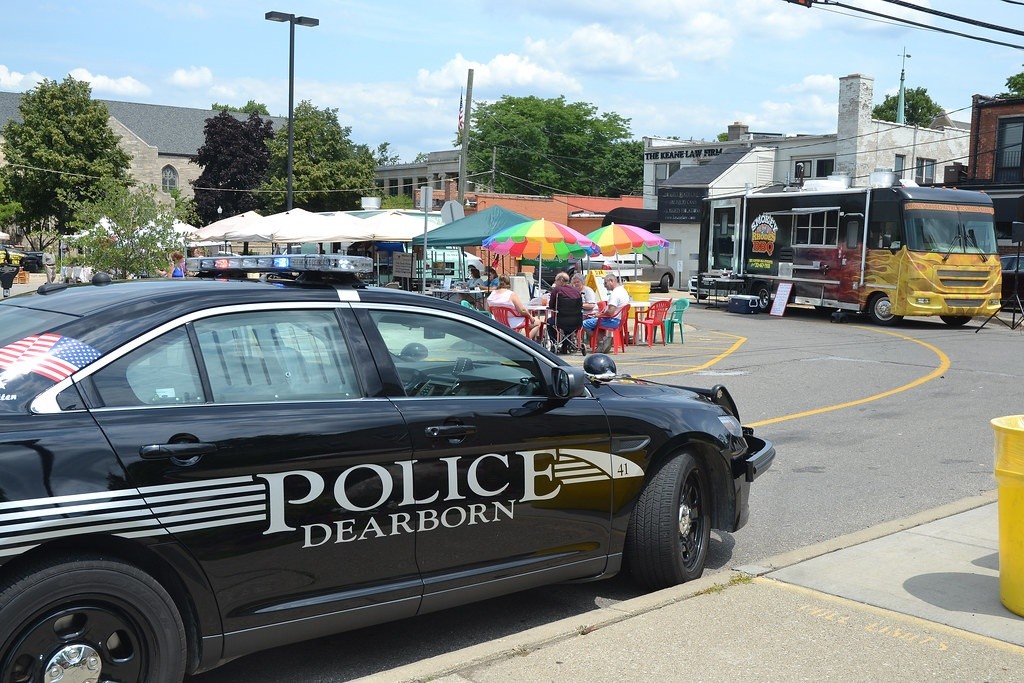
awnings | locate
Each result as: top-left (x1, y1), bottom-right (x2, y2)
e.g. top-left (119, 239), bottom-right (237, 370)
top-left (763, 209), bottom-right (837, 215)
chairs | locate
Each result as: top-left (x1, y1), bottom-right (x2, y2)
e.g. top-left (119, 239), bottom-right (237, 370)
top-left (461, 299), bottom-right (554, 345)
top-left (577, 297), bottom-right (690, 354)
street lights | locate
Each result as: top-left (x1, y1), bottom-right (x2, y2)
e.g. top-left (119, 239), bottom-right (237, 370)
top-left (262, 11), bottom-right (319, 211)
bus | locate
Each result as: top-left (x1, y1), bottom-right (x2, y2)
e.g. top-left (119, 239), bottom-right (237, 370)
top-left (699, 182), bottom-right (1024, 326)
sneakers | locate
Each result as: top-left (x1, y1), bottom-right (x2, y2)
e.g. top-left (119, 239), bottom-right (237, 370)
top-left (602, 337), bottom-right (612, 353)
top-left (596, 343), bottom-right (603, 353)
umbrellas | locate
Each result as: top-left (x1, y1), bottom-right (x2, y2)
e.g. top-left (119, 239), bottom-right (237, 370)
top-left (583, 222), bottom-right (669, 286)
top-left (482, 217), bottom-right (601, 320)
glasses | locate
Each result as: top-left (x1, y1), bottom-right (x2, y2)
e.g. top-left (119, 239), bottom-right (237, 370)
top-left (603, 280), bottom-right (610, 285)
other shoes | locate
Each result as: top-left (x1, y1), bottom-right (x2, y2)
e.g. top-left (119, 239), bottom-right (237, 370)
top-left (560, 347), bottom-right (567, 353)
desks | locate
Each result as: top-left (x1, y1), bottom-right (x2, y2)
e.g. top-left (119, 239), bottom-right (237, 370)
top-left (430, 288), bottom-right (494, 311)
top-left (525, 305), bottom-right (553, 344)
top-left (630, 301), bottom-right (651, 343)
top-left (703, 278), bottom-right (745, 311)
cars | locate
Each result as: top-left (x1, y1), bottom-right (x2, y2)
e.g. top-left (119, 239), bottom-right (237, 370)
top-left (687, 274), bottom-right (737, 299)
top-left (1, 254), bottom-right (777, 683)
top-left (532, 251), bottom-right (675, 293)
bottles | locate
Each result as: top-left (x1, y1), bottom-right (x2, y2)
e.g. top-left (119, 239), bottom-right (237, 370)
top-left (581, 292), bottom-right (586, 305)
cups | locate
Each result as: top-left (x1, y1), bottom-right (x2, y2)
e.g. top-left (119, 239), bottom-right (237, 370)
top-left (541, 297), bottom-right (547, 306)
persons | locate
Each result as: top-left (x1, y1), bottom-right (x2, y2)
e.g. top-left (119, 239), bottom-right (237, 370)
top-left (479, 268), bottom-right (499, 310)
top-left (570, 273), bottom-right (601, 340)
top-left (547, 271), bottom-right (581, 355)
top-left (42, 246), bottom-right (57, 284)
top-left (462, 268), bottom-right (485, 300)
top-left (153, 248), bottom-right (208, 278)
top-left (583, 274), bottom-right (629, 354)
top-left (487, 274), bottom-right (541, 340)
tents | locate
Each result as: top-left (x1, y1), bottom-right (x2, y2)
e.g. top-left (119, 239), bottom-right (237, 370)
top-left (409, 204), bottom-right (536, 293)
top-left (116, 212), bottom-right (232, 280)
top-left (184, 206), bottom-right (444, 287)
top-left (60, 217), bottom-right (130, 283)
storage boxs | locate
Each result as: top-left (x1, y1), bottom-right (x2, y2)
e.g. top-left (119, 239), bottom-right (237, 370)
top-left (728, 295), bottom-right (761, 313)
